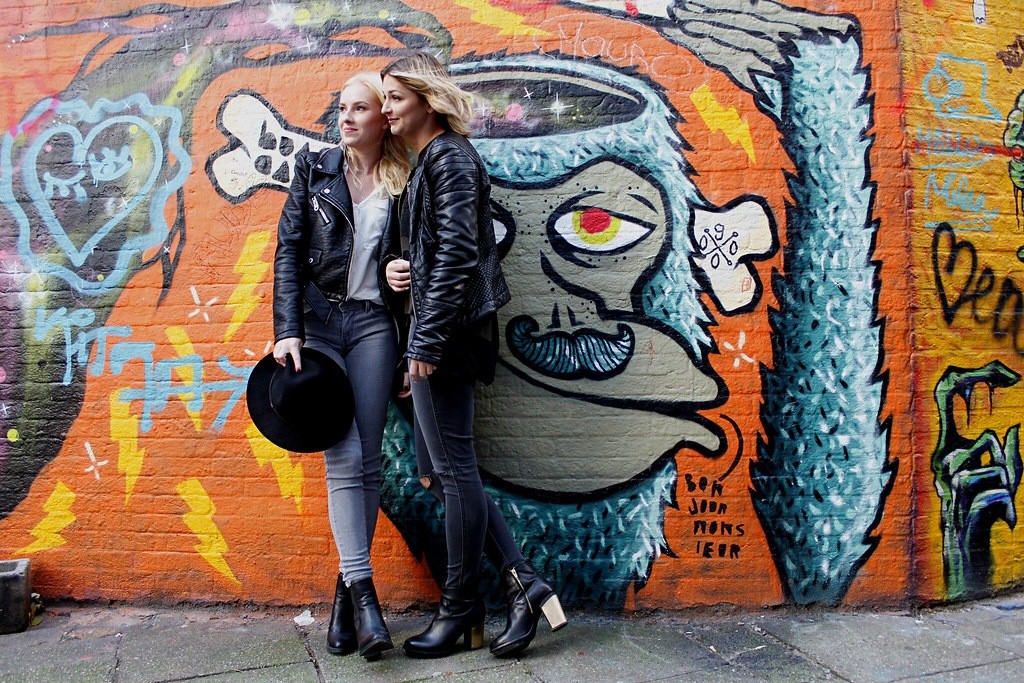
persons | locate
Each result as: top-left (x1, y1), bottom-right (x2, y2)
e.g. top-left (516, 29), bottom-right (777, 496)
top-left (272, 70), bottom-right (412, 660)
top-left (377, 52), bottom-right (567, 658)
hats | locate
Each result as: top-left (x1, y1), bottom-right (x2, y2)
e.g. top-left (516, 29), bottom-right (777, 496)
top-left (245, 346), bottom-right (357, 453)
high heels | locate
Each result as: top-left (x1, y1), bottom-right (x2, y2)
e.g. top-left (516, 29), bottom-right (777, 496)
top-left (490, 558), bottom-right (569, 655)
top-left (327, 572), bottom-right (395, 656)
top-left (403, 585), bottom-right (484, 657)
